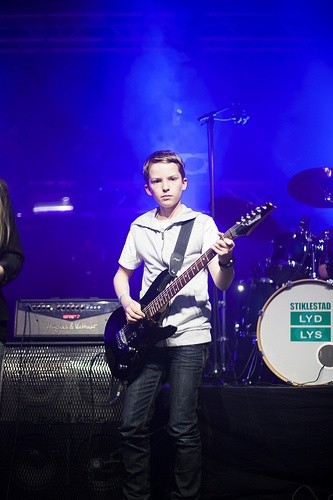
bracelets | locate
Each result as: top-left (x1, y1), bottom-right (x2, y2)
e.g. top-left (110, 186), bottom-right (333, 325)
top-left (117, 292), bottom-right (131, 304)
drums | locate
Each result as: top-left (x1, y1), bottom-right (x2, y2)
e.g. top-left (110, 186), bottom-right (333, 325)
top-left (259, 256), bottom-right (312, 288)
top-left (226, 275), bottom-right (276, 337)
top-left (254, 277), bottom-right (333, 386)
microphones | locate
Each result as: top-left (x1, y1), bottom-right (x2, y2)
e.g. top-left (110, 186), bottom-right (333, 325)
top-left (239, 104), bottom-right (248, 124)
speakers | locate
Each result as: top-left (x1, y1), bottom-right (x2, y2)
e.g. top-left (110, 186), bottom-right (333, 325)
top-left (0, 340), bottom-right (130, 500)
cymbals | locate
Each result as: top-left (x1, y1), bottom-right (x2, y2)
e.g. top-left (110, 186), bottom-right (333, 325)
top-left (287, 167), bottom-right (333, 208)
top-left (277, 226), bottom-right (317, 244)
top-left (208, 196), bottom-right (275, 239)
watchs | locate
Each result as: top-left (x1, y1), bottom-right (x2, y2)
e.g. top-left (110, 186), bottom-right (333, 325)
top-left (218, 256), bottom-right (235, 269)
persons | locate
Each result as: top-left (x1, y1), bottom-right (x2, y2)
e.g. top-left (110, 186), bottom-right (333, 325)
top-left (0, 175), bottom-right (28, 391)
top-left (112, 148), bottom-right (236, 500)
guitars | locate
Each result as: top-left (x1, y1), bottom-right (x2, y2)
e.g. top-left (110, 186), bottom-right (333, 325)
top-left (102, 200), bottom-right (279, 381)
